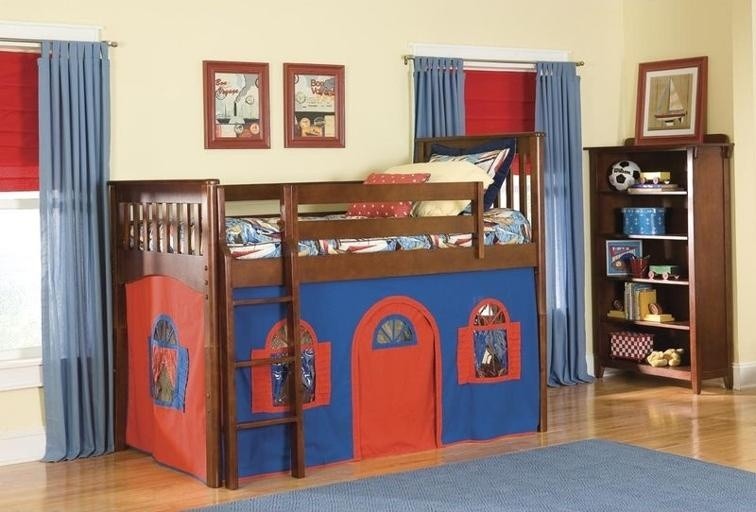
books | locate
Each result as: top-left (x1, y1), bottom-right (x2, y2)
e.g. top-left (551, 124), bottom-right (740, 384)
top-left (623, 282), bottom-right (656, 321)
top-left (627, 187), bottom-right (685, 191)
top-left (632, 183), bottom-right (680, 188)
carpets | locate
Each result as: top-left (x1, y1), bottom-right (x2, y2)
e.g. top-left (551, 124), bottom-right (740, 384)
top-left (180, 437), bottom-right (755, 512)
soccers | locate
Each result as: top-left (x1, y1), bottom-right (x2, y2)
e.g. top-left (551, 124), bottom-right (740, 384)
top-left (609, 160), bottom-right (640, 190)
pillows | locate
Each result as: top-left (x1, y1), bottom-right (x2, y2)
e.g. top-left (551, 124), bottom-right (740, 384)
top-left (344, 135), bottom-right (519, 231)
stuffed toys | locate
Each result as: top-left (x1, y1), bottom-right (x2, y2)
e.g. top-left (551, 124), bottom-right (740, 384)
top-left (647, 346), bottom-right (684, 367)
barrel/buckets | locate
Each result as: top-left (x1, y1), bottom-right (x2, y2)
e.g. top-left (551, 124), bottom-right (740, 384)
top-left (627, 257), bottom-right (648, 277)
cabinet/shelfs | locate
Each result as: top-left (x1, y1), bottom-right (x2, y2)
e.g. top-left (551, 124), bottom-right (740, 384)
top-left (583, 148), bottom-right (737, 396)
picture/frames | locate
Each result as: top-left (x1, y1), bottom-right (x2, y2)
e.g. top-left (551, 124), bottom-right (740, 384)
top-left (632, 54), bottom-right (711, 149)
top-left (198, 58), bottom-right (349, 149)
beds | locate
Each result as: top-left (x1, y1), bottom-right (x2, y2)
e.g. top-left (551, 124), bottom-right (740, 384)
top-left (102, 130), bottom-right (550, 488)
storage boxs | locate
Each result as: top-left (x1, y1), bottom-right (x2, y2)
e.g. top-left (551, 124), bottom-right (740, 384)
top-left (609, 328), bottom-right (657, 364)
top-left (621, 206), bottom-right (670, 238)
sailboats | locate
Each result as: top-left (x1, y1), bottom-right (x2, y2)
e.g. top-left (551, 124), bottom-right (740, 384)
top-left (655, 77), bottom-right (689, 126)
